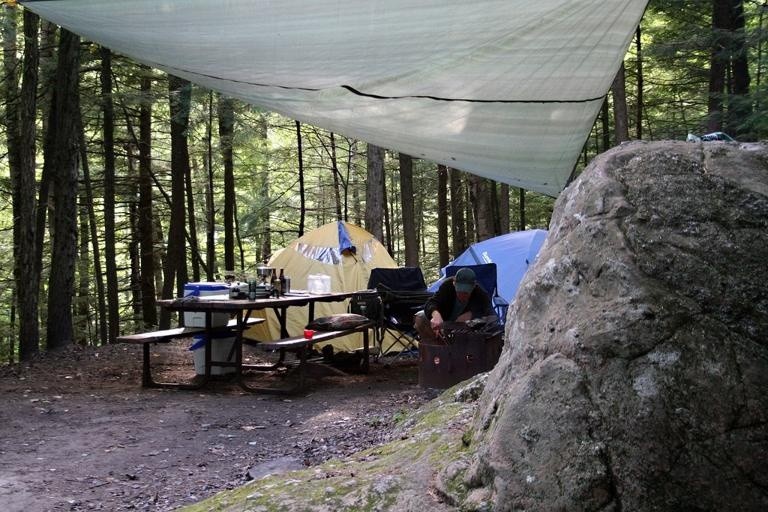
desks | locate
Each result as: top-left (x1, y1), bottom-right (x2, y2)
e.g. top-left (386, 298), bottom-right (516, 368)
top-left (154, 289), bottom-right (353, 396)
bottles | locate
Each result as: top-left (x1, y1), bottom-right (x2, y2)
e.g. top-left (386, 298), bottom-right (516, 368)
top-left (279, 268), bottom-right (286, 293)
top-left (248, 280), bottom-right (257, 302)
top-left (270, 269), bottom-right (277, 295)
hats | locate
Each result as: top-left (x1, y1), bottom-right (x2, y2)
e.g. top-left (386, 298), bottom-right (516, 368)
top-left (455, 268), bottom-right (476, 292)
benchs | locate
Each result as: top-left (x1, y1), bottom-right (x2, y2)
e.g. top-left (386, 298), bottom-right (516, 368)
top-left (115, 316), bottom-right (265, 389)
top-left (257, 320), bottom-right (378, 396)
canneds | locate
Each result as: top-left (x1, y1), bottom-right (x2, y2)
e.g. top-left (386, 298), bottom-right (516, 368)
top-left (274, 279), bottom-right (282, 296)
top-left (249, 280), bottom-right (256, 300)
top-left (284, 277), bottom-right (290, 294)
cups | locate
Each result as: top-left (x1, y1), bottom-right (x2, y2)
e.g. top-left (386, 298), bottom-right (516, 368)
top-left (303, 329), bottom-right (314, 339)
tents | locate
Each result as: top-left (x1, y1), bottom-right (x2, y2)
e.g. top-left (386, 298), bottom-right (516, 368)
top-left (425, 229), bottom-right (551, 326)
top-left (227, 222), bottom-right (424, 356)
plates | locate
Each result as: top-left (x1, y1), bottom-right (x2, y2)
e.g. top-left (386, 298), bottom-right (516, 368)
top-left (238, 285), bottom-right (273, 298)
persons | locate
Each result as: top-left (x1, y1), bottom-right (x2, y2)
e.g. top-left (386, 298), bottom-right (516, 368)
top-left (413, 268), bottom-right (497, 343)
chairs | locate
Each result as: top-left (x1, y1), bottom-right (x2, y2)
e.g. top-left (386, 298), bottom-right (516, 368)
top-left (367, 262), bottom-right (509, 367)
